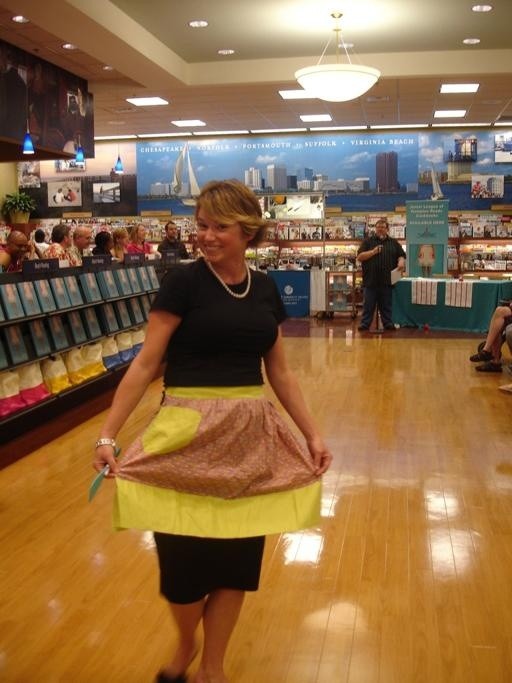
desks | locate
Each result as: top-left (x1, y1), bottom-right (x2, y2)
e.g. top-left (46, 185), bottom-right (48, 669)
top-left (262, 265), bottom-right (325, 320)
top-left (389, 273), bottom-right (512, 334)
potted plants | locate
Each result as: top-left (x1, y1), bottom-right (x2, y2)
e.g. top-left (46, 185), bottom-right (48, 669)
top-left (0, 192), bottom-right (37, 224)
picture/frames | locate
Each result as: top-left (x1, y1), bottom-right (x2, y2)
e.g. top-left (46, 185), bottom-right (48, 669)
top-left (91, 181), bottom-right (121, 205)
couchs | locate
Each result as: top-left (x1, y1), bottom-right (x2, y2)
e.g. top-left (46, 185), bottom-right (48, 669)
top-left (505, 322), bottom-right (512, 354)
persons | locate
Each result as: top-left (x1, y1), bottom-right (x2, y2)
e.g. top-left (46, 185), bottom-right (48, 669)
top-left (357, 220), bottom-right (406, 331)
top-left (0, 220), bottom-right (191, 272)
top-left (93, 178), bottom-right (333, 682)
top-left (470, 299), bottom-right (512, 372)
top-left (498, 323), bottom-right (512, 392)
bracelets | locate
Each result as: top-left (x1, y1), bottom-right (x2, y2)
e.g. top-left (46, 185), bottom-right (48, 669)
top-left (93, 438), bottom-right (116, 450)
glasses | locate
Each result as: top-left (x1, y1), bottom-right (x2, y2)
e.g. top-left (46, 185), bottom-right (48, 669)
top-left (10, 241), bottom-right (28, 249)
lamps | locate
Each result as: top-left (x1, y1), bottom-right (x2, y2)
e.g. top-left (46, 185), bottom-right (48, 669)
top-left (21, 52), bottom-right (35, 156)
top-left (113, 93), bottom-right (126, 175)
top-left (293, 10), bottom-right (382, 104)
top-left (74, 75), bottom-right (85, 165)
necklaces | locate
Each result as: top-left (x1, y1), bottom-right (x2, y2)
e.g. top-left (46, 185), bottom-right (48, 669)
top-left (202, 258), bottom-right (251, 298)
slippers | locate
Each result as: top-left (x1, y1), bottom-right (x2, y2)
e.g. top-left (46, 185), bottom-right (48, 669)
top-left (155, 666), bottom-right (192, 682)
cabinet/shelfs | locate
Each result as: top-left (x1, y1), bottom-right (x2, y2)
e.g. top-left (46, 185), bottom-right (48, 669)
top-left (0, 250), bottom-right (178, 447)
top-left (323, 268), bottom-right (360, 320)
top-left (324, 212), bottom-right (366, 307)
top-left (256, 219), bottom-right (324, 271)
top-left (459, 214), bottom-right (512, 273)
top-left (366, 212), bottom-right (459, 273)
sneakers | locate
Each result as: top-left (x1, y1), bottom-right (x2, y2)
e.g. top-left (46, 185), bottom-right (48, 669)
top-left (358, 325), bottom-right (366, 332)
top-left (499, 383), bottom-right (512, 396)
top-left (475, 363), bottom-right (503, 374)
top-left (385, 324), bottom-right (395, 331)
top-left (469, 350), bottom-right (493, 361)
